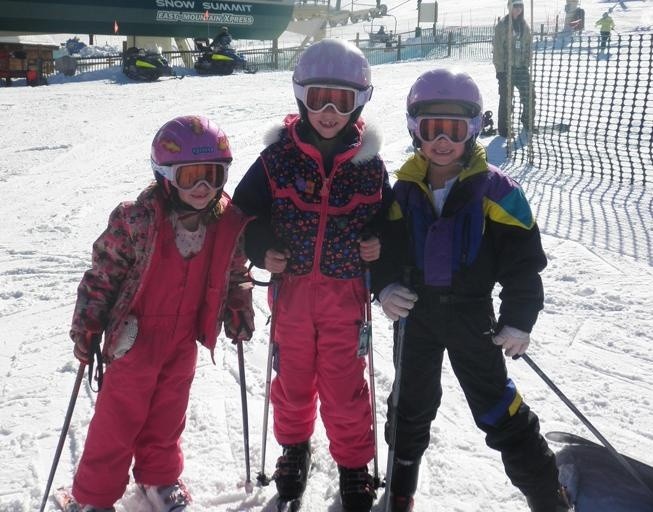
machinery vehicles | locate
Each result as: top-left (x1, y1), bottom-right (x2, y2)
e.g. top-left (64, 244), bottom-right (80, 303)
top-left (119, 39), bottom-right (257, 82)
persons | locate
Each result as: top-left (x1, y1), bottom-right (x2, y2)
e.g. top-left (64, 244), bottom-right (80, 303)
top-left (232, 38), bottom-right (391, 512)
top-left (371, 68), bottom-right (578, 512)
top-left (492, 0), bottom-right (541, 137)
top-left (69, 115), bottom-right (254, 512)
top-left (595, 12), bottom-right (614, 49)
top-left (210, 27), bottom-right (231, 51)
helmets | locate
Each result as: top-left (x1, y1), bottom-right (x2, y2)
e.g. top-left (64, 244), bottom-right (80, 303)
top-left (152, 115), bottom-right (231, 197)
top-left (293, 39), bottom-right (370, 120)
top-left (407, 69), bottom-right (482, 116)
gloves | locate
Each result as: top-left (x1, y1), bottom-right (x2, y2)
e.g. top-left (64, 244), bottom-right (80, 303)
top-left (379, 283), bottom-right (418, 321)
top-left (492, 325), bottom-right (530, 356)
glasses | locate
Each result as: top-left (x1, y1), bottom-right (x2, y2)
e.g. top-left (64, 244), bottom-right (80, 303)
top-left (407, 114), bottom-right (482, 144)
top-left (294, 83), bottom-right (371, 116)
top-left (151, 157), bottom-right (228, 190)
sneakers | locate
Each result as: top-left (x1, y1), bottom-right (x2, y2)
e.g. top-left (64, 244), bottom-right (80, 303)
top-left (527, 490), bottom-right (574, 512)
top-left (389, 494), bottom-right (414, 512)
top-left (338, 465), bottom-right (372, 511)
top-left (147, 479), bottom-right (192, 511)
top-left (275, 442), bottom-right (310, 497)
